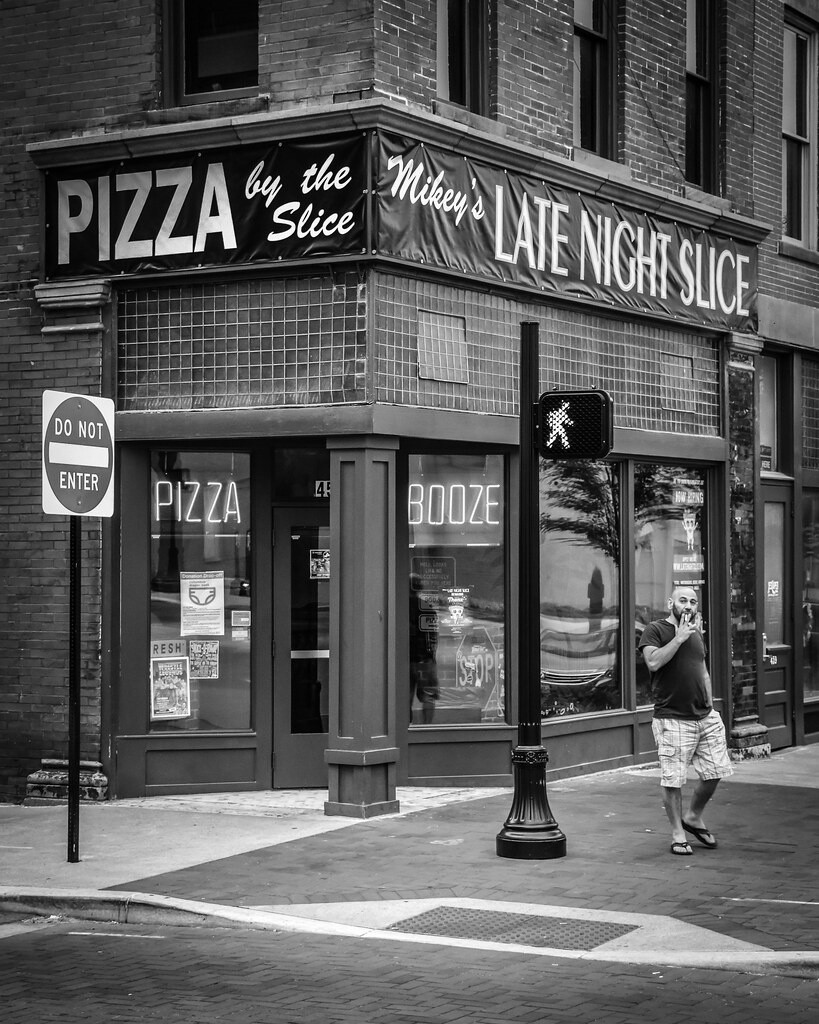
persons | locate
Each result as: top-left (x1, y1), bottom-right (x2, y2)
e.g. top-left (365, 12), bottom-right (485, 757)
top-left (638, 588), bottom-right (732, 856)
top-left (408, 573), bottom-right (440, 725)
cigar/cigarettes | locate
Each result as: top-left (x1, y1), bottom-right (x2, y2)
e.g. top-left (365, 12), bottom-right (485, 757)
top-left (685, 614), bottom-right (687, 622)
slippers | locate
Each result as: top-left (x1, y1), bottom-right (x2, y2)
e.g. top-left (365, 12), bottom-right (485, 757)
top-left (670, 841), bottom-right (693, 855)
top-left (681, 818), bottom-right (718, 848)
